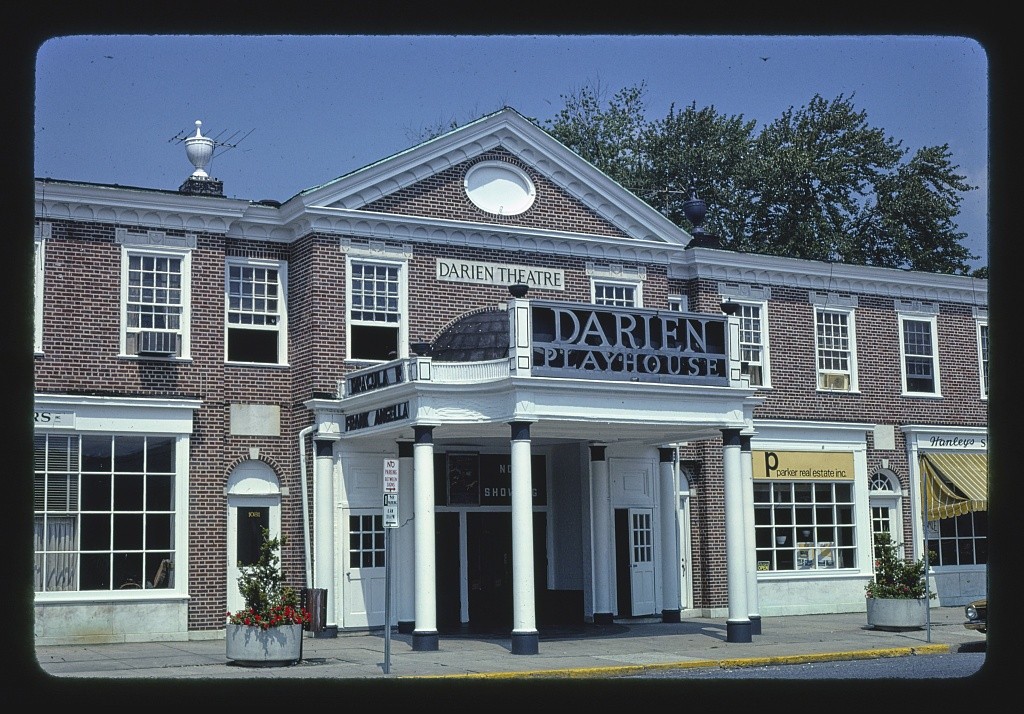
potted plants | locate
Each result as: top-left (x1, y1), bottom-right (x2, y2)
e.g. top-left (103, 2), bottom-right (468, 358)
top-left (863, 533), bottom-right (939, 631)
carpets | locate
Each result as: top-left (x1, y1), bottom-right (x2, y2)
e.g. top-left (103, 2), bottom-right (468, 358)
top-left (490, 622), bottom-right (629, 638)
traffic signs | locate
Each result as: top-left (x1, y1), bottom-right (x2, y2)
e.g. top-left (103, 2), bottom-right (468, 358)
top-left (383, 458), bottom-right (400, 493)
top-left (383, 493), bottom-right (399, 529)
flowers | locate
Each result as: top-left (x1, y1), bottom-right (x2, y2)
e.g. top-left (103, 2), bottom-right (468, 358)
top-left (224, 525), bottom-right (310, 630)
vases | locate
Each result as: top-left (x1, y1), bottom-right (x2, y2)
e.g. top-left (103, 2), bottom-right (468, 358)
top-left (225, 624), bottom-right (304, 665)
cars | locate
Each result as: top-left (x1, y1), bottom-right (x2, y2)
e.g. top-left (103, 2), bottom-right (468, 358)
top-left (962, 599), bottom-right (987, 633)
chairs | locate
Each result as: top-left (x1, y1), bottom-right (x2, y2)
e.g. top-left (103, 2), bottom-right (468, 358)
top-left (119, 559), bottom-right (172, 589)
